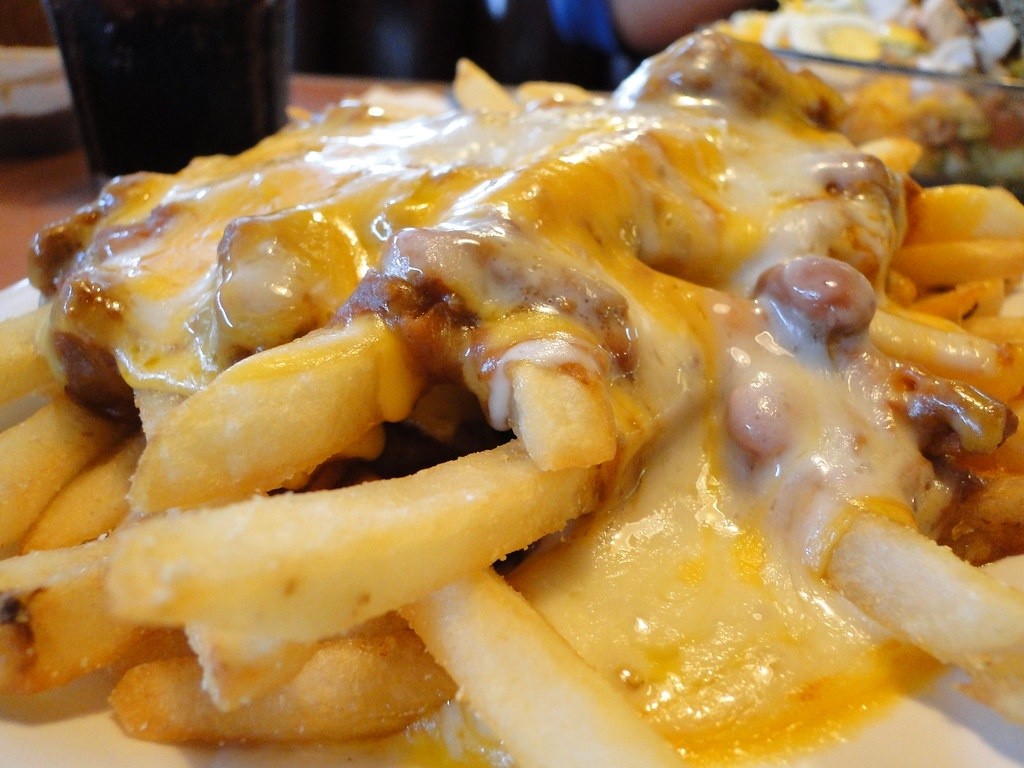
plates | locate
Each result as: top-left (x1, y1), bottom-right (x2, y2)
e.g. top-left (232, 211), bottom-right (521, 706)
top-left (0, 277), bottom-right (1024, 767)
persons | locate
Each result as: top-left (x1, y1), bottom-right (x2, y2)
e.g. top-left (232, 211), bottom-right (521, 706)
top-left (295, 0), bottom-right (757, 93)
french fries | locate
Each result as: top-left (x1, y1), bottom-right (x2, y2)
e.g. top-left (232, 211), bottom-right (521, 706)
top-left (1, 157), bottom-right (1024, 768)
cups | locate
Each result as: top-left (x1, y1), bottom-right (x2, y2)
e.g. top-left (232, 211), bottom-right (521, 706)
top-left (40, 1), bottom-right (297, 200)
